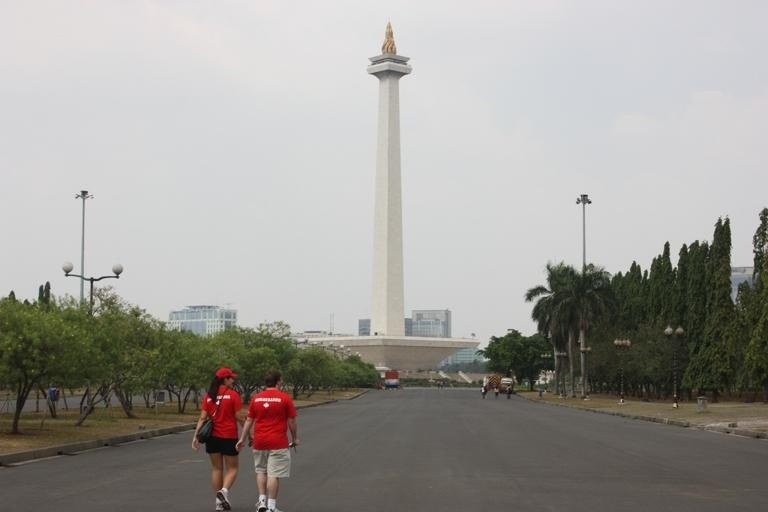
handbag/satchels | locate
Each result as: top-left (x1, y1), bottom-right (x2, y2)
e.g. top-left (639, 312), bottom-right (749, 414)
top-left (196, 418), bottom-right (213, 444)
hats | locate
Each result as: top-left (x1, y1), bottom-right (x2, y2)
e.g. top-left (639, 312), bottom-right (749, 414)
top-left (215, 367), bottom-right (238, 382)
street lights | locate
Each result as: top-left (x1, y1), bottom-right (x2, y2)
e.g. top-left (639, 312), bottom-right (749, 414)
top-left (665, 324), bottom-right (689, 409)
top-left (613, 337), bottom-right (631, 407)
top-left (62, 262), bottom-right (123, 321)
top-left (75, 187), bottom-right (95, 316)
top-left (540, 344), bottom-right (597, 400)
top-left (574, 191), bottom-right (595, 394)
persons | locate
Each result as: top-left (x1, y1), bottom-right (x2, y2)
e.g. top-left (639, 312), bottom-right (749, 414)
top-left (480, 385), bottom-right (486, 399)
top-left (493, 385), bottom-right (500, 399)
top-left (504, 383), bottom-right (513, 398)
top-left (234, 368), bottom-right (301, 511)
top-left (190, 365), bottom-right (246, 511)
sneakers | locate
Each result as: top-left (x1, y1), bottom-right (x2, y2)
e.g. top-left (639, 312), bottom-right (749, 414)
top-left (215, 488), bottom-right (231, 511)
top-left (256, 499), bottom-right (284, 512)
